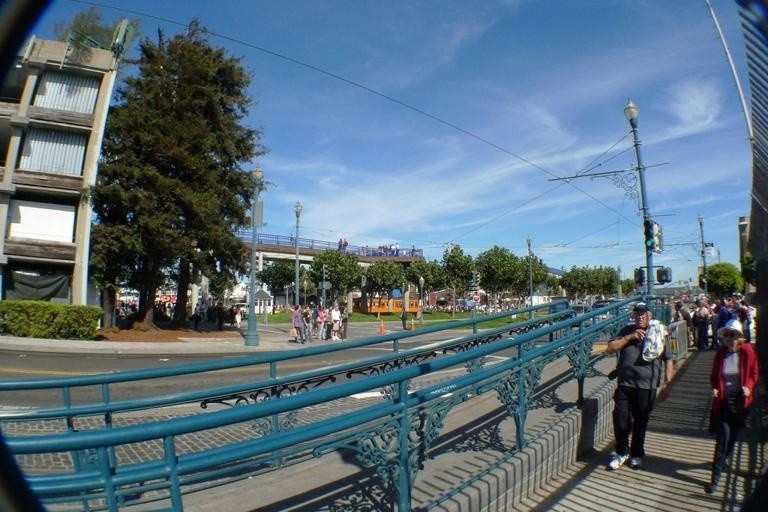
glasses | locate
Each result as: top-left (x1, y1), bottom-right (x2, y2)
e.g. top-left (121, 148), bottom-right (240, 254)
top-left (722, 332), bottom-right (736, 339)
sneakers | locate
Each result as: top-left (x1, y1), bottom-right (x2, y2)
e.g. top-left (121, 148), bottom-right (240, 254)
top-left (608, 453), bottom-right (630, 470)
top-left (630, 456), bottom-right (642, 468)
top-left (707, 475), bottom-right (719, 495)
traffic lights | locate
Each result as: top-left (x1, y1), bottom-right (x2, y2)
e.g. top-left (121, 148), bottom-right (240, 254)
top-left (704, 265), bottom-right (708, 278)
top-left (643, 220), bottom-right (654, 249)
top-left (468, 271), bottom-right (480, 282)
top-left (634, 269), bottom-right (645, 284)
top-left (657, 267), bottom-right (672, 282)
top-left (325, 264), bottom-right (329, 280)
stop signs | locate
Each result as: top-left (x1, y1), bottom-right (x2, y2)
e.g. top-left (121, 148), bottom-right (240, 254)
top-left (473, 291), bottom-right (480, 303)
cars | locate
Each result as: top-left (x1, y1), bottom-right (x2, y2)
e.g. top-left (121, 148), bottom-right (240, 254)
top-left (236, 303), bottom-right (249, 315)
top-left (120, 292), bottom-right (140, 301)
top-left (548, 299), bottom-right (622, 326)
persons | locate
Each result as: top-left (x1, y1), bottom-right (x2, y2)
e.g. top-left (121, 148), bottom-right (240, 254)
top-left (193, 303), bottom-right (242, 331)
top-left (673, 292), bottom-right (753, 350)
top-left (288, 302), bottom-right (349, 344)
top-left (120, 299), bottom-right (176, 319)
top-left (364, 245), bottom-right (369, 256)
top-left (606, 301), bottom-right (673, 471)
top-left (342, 238), bottom-right (348, 252)
top-left (705, 319), bottom-right (759, 493)
top-left (338, 238), bottom-right (343, 251)
top-left (402, 248), bottom-right (406, 256)
top-left (400, 308), bottom-right (407, 329)
top-left (411, 245), bottom-right (415, 256)
top-left (378, 242), bottom-right (400, 256)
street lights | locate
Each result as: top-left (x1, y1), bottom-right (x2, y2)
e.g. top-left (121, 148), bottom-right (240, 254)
top-left (244, 161), bottom-right (264, 347)
top-left (526, 235), bottom-right (534, 319)
top-left (547, 97), bottom-right (676, 314)
top-left (697, 213), bottom-right (714, 291)
top-left (419, 276), bottom-right (425, 324)
top-left (293, 199), bottom-right (307, 305)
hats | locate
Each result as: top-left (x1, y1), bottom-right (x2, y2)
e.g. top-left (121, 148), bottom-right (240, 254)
top-left (717, 318), bottom-right (744, 338)
top-left (632, 302), bottom-right (648, 311)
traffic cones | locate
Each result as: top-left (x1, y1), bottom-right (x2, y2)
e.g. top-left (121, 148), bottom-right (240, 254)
top-left (380, 319), bottom-right (384, 336)
top-left (411, 318), bottom-right (415, 330)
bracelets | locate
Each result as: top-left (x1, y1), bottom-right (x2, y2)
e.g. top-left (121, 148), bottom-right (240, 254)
top-left (665, 381), bottom-right (671, 385)
